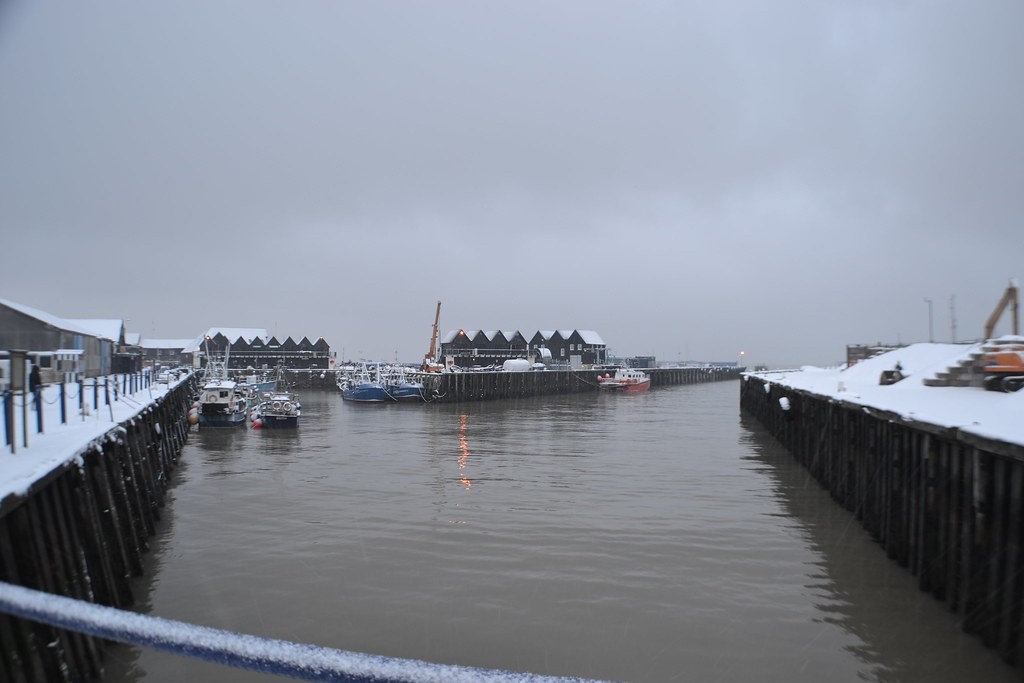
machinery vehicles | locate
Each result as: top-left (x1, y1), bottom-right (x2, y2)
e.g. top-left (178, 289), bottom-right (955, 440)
top-left (981, 286), bottom-right (1024, 394)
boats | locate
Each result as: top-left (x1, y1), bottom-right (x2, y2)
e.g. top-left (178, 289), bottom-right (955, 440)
top-left (249, 363), bottom-right (302, 430)
top-left (597, 367), bottom-right (651, 392)
top-left (342, 362), bottom-right (385, 402)
top-left (380, 377), bottom-right (424, 403)
top-left (186, 340), bottom-right (250, 428)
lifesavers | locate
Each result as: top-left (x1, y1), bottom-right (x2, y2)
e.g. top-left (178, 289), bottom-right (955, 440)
top-left (282, 402), bottom-right (292, 412)
top-left (272, 401), bottom-right (281, 411)
top-left (209, 395), bottom-right (218, 403)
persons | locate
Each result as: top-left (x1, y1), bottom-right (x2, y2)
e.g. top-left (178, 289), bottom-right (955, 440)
top-left (28, 364), bottom-right (42, 411)
